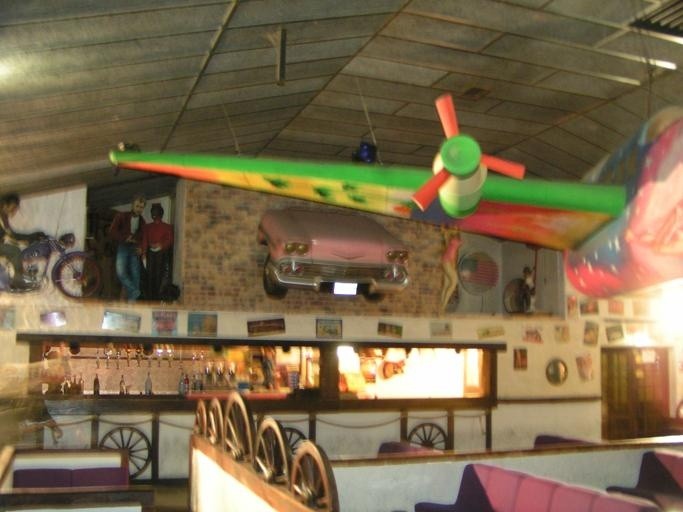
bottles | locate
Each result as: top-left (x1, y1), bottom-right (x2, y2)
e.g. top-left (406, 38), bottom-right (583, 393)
top-left (71, 372), bottom-right (189, 396)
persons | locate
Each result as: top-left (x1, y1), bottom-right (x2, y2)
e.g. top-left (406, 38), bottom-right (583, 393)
top-left (110, 196), bottom-right (146, 300)
top-left (141, 203), bottom-right (174, 300)
top-left (0, 192), bottom-right (46, 290)
